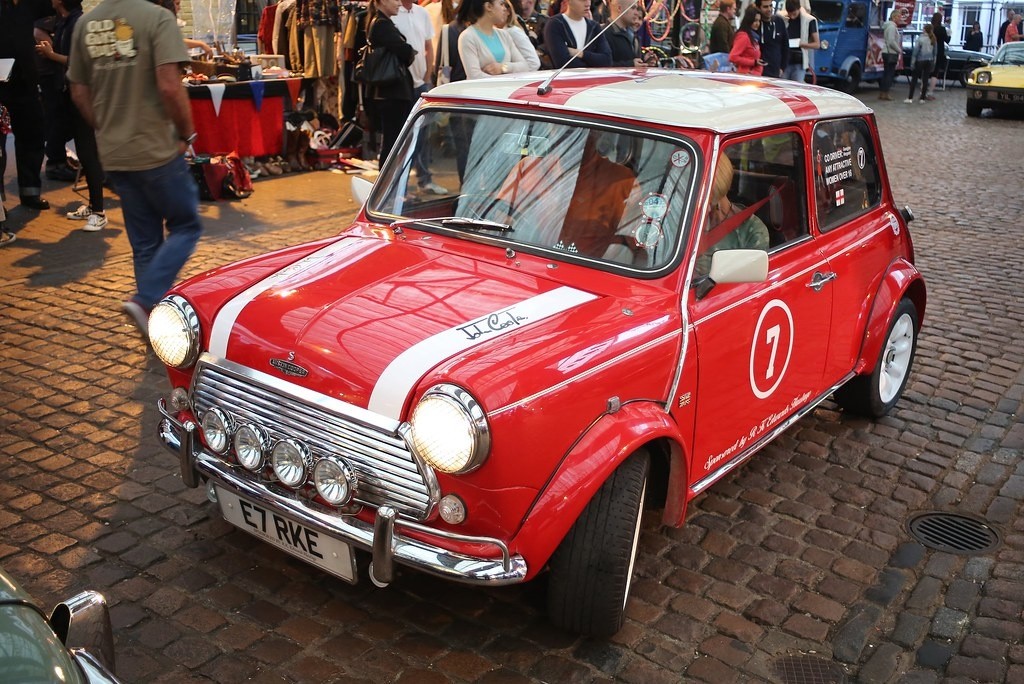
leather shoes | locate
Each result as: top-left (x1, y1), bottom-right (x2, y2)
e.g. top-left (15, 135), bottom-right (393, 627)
top-left (23, 199), bottom-right (49, 208)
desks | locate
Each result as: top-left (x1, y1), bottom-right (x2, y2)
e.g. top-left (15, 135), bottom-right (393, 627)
top-left (188, 77), bottom-right (321, 157)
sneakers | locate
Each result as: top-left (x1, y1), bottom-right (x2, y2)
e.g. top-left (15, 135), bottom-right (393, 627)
top-left (67, 205), bottom-right (94, 219)
top-left (83, 211), bottom-right (107, 231)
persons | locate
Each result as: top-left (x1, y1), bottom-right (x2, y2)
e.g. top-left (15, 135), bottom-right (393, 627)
top-left (486, 120), bottom-right (644, 262)
top-left (65, 0), bottom-right (204, 342)
top-left (944, 17), bottom-right (951, 29)
top-left (386, 0), bottom-right (452, 197)
top-left (902, 24), bottom-right (936, 103)
top-left (364, 0), bottom-right (423, 207)
top-left (619, 144), bottom-right (772, 282)
top-left (161, 0), bottom-right (214, 59)
top-left (0, 0), bottom-right (108, 246)
top-left (966, 21), bottom-right (984, 52)
top-left (709, 0), bottom-right (822, 82)
top-left (432, 0), bottom-right (658, 198)
top-left (927, 13), bottom-right (951, 100)
top-left (879, 9), bottom-right (904, 101)
top-left (995, 8), bottom-right (1024, 49)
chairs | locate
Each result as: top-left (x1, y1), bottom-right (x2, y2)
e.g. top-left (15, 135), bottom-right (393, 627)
top-left (729, 170), bottom-right (789, 248)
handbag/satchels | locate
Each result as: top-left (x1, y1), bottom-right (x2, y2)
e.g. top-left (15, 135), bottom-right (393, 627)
top-left (351, 18), bottom-right (408, 82)
top-left (883, 53), bottom-right (898, 65)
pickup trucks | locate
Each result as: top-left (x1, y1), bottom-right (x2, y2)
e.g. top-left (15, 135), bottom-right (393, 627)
top-left (805, 0), bottom-right (904, 94)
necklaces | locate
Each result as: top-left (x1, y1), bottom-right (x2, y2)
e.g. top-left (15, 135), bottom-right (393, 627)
top-left (721, 202), bottom-right (733, 224)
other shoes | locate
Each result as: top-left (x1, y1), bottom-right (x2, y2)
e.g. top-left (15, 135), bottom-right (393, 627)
top-left (919, 100), bottom-right (926, 104)
top-left (125, 301), bottom-right (150, 341)
top-left (903, 98), bottom-right (913, 104)
top-left (0, 233), bottom-right (15, 245)
top-left (417, 183), bottom-right (448, 195)
top-left (880, 93), bottom-right (893, 101)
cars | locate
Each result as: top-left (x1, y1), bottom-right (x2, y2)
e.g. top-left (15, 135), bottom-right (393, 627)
top-left (896, 30), bottom-right (1008, 89)
top-left (147, 67), bottom-right (928, 641)
top-left (966, 41), bottom-right (1024, 117)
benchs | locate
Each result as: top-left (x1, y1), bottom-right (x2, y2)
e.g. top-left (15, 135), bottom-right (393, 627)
top-left (811, 180), bottom-right (867, 228)
